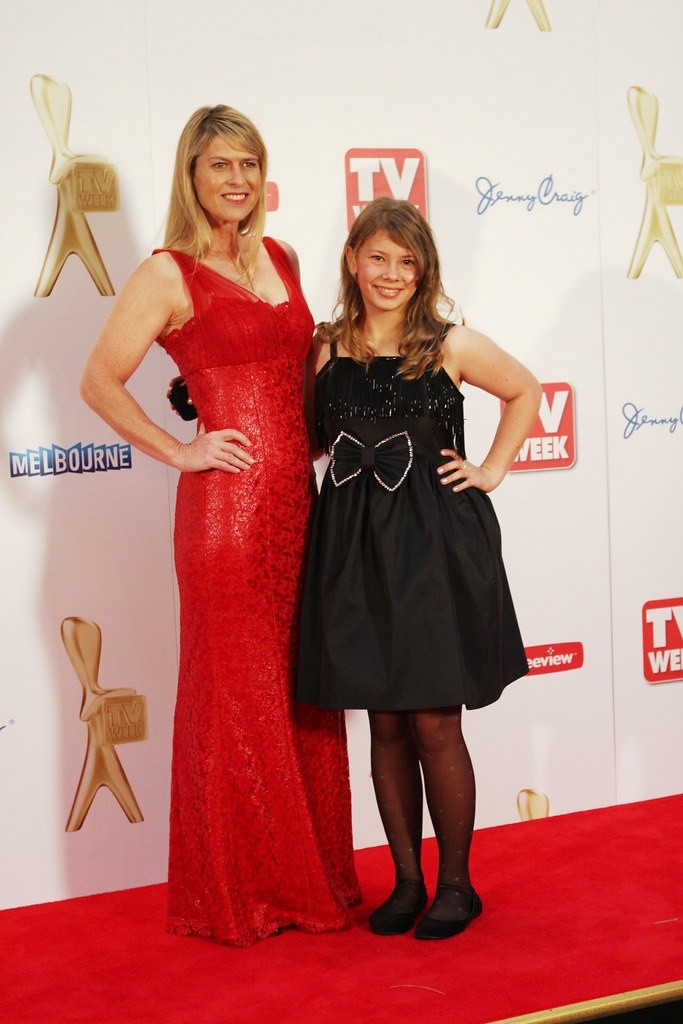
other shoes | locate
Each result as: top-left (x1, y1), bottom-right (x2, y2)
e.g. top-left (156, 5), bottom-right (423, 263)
top-left (369, 884), bottom-right (428, 935)
top-left (415, 886), bottom-right (482, 940)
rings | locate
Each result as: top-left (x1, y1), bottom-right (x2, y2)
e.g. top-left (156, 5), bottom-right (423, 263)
top-left (461, 462), bottom-right (466, 470)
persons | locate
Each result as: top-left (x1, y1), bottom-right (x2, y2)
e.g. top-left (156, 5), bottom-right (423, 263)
top-left (163, 196), bottom-right (542, 947)
top-left (74, 102), bottom-right (371, 945)
